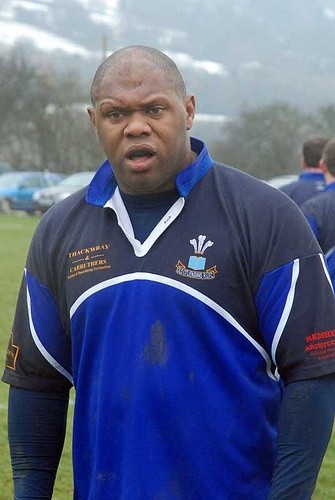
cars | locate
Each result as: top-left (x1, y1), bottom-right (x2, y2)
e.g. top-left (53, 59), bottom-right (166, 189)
top-left (2, 171), bottom-right (96, 216)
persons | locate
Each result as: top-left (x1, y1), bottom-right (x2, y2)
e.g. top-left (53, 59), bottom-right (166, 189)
top-left (0, 45), bottom-right (335, 500)
top-left (278, 137), bottom-right (328, 207)
top-left (300, 140), bottom-right (335, 292)
